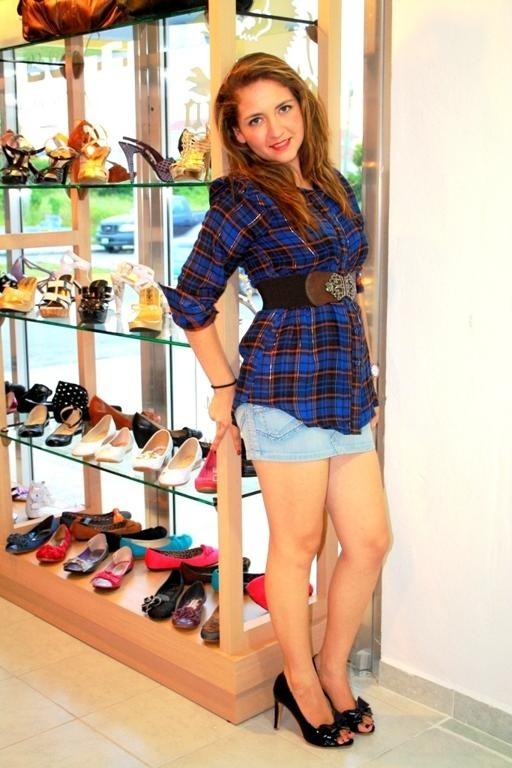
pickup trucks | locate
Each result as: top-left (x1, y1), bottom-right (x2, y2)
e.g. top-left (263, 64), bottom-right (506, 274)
top-left (97, 195), bottom-right (208, 252)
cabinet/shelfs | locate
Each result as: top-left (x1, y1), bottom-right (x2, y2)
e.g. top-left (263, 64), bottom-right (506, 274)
top-left (0, 1), bottom-right (390, 727)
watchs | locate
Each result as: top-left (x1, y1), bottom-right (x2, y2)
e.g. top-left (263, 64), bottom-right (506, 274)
top-left (369, 362), bottom-right (382, 376)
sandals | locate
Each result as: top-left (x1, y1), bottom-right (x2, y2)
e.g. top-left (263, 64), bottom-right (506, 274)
top-left (89, 546), bottom-right (135, 591)
top-left (25, 481), bottom-right (59, 519)
top-left (5, 515), bottom-right (59, 554)
top-left (63, 533), bottom-right (110, 574)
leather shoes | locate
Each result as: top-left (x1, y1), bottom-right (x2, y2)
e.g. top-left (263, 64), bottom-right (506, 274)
top-left (103, 526), bottom-right (167, 551)
top-left (142, 568), bottom-right (186, 620)
top-left (18, 405), bottom-right (50, 437)
top-left (72, 414), bottom-right (116, 456)
top-left (53, 381), bottom-right (122, 422)
top-left (96, 426), bottom-right (133, 462)
top-left (36, 523), bottom-right (72, 563)
top-left (72, 519), bottom-right (142, 542)
top-left (119, 535), bottom-right (193, 559)
top-left (60, 510), bottom-right (131, 527)
top-left (201, 605), bottom-right (220, 642)
top-left (143, 544), bottom-right (219, 571)
top-left (183, 557), bottom-right (251, 583)
top-left (171, 581), bottom-right (207, 629)
top-left (211, 569), bottom-right (265, 592)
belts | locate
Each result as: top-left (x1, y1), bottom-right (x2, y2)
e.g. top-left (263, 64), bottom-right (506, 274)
top-left (256, 271), bottom-right (356, 308)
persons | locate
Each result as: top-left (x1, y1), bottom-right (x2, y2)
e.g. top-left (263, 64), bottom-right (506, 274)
top-left (158, 53), bottom-right (394, 750)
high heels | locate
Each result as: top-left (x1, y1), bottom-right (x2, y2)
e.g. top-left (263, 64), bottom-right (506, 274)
top-left (0, 277), bottom-right (37, 313)
top-left (39, 273), bottom-right (73, 317)
top-left (159, 438), bottom-right (203, 486)
top-left (129, 286), bottom-right (163, 332)
top-left (0, 136), bottom-right (45, 184)
top-left (119, 136), bottom-right (177, 182)
top-left (114, 263), bottom-right (171, 312)
top-left (272, 671), bottom-right (354, 747)
top-left (78, 279), bottom-right (112, 323)
top-left (36, 146), bottom-right (81, 184)
top-left (46, 405), bottom-right (84, 446)
top-left (59, 249), bottom-right (115, 301)
top-left (195, 449), bottom-right (216, 492)
top-left (132, 413), bottom-right (203, 448)
top-left (171, 130), bottom-right (211, 180)
top-left (134, 429), bottom-right (173, 472)
top-left (10, 255), bottom-right (57, 295)
top-left (76, 138), bottom-right (112, 184)
top-left (312, 654), bottom-right (375, 734)
top-left (68, 120), bottom-right (137, 181)
top-left (89, 395), bottom-right (162, 430)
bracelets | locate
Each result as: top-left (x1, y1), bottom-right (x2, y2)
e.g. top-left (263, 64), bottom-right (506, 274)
top-left (208, 380), bottom-right (237, 393)
